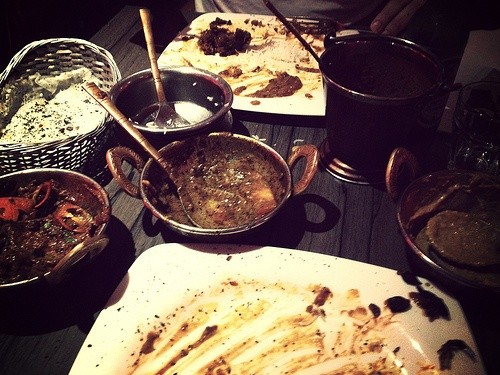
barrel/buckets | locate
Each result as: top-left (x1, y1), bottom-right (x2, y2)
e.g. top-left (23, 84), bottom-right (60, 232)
top-left (317, 31), bottom-right (465, 185)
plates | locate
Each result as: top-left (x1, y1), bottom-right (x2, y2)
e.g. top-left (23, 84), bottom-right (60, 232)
top-left (151, 11), bottom-right (331, 116)
top-left (66, 241), bottom-right (490, 374)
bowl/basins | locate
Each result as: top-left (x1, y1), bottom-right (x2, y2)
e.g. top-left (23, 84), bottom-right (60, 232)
top-left (0, 168), bottom-right (111, 288)
top-left (103, 67), bottom-right (234, 144)
top-left (105, 130), bottom-right (319, 244)
top-left (385, 146), bottom-right (500, 298)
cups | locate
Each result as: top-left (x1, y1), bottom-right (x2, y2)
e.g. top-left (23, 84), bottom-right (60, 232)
top-left (452, 79), bottom-right (500, 172)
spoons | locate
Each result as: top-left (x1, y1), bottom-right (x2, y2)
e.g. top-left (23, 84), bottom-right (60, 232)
top-left (82, 82), bottom-right (247, 228)
top-left (139, 8), bottom-right (190, 128)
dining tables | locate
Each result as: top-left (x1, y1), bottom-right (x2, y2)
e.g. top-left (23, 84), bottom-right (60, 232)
top-left (0, 0), bottom-right (500, 375)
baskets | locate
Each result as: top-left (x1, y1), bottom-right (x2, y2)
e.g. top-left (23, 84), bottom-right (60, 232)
top-left (0, 37), bottom-right (122, 176)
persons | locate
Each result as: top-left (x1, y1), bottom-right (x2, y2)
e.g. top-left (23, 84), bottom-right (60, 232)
top-left (194, 0), bottom-right (427, 36)
